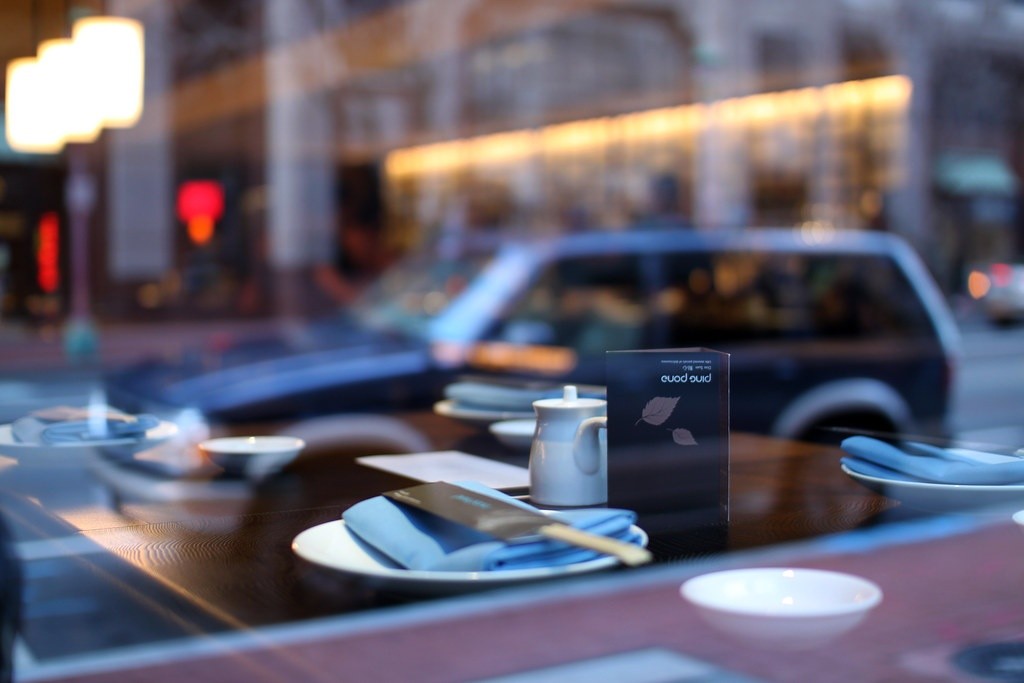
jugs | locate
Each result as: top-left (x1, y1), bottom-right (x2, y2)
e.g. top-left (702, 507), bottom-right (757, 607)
top-left (524, 382), bottom-right (608, 508)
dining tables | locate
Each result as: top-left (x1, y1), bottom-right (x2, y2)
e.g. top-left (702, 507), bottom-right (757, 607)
top-left (0, 412), bottom-right (1024, 682)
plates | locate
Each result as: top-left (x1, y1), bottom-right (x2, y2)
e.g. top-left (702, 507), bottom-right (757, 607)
top-left (0, 417), bottom-right (178, 468)
top-left (287, 493), bottom-right (649, 592)
top-left (429, 386), bottom-right (617, 428)
top-left (841, 446), bottom-right (1024, 517)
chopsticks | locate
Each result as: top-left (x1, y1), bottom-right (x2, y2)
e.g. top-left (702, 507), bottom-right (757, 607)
top-left (531, 520), bottom-right (653, 567)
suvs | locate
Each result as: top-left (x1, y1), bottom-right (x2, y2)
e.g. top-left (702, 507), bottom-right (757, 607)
top-left (84, 226), bottom-right (970, 563)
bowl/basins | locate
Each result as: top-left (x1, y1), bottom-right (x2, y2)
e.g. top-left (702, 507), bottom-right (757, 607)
top-left (197, 434), bottom-right (306, 477)
top-left (678, 567), bottom-right (884, 651)
top-left (488, 419), bottom-right (543, 451)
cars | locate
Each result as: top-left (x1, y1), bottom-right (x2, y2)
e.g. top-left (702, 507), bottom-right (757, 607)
top-left (979, 263), bottom-right (1024, 328)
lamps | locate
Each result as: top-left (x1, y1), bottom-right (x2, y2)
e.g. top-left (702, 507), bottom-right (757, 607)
top-left (4, 0), bottom-right (145, 155)
